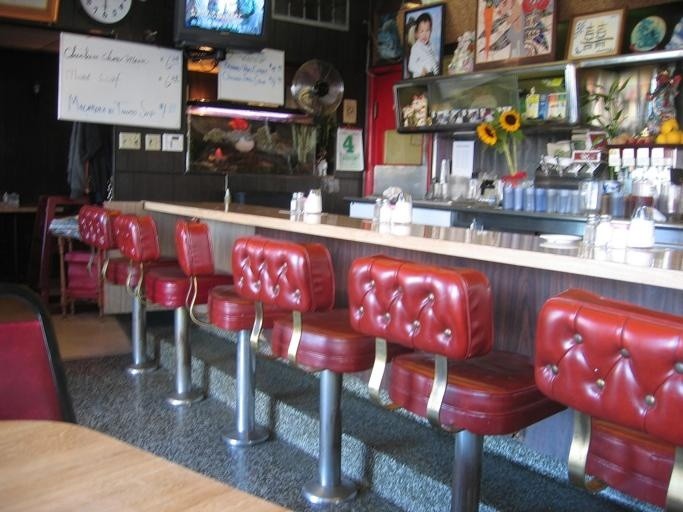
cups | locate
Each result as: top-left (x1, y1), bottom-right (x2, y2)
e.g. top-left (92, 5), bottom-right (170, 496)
top-left (630, 179), bottom-right (679, 218)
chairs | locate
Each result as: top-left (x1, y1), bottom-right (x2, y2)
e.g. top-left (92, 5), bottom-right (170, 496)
top-left (34, 194), bottom-right (91, 314)
top-left (0, 312), bottom-right (68, 423)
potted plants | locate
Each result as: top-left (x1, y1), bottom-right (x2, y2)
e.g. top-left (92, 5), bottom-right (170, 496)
top-left (579, 75), bottom-right (631, 144)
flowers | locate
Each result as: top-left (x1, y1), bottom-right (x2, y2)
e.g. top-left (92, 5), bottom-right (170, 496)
top-left (473, 107), bottom-right (524, 183)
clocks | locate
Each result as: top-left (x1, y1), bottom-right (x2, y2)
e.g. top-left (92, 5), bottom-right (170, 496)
top-left (78, 0), bottom-right (133, 25)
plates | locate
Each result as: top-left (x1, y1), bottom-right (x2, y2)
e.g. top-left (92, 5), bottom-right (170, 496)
top-left (538, 232), bottom-right (580, 245)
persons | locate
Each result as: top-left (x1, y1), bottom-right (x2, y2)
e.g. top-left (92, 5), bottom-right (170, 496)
top-left (406, 13), bottom-right (438, 78)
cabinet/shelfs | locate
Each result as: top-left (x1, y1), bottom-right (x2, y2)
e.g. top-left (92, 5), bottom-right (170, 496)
top-left (345, 48), bottom-right (683, 247)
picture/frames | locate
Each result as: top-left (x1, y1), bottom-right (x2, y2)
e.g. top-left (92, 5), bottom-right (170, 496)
top-left (473, 0), bottom-right (556, 71)
top-left (562, 4), bottom-right (628, 60)
top-left (0, 0), bottom-right (59, 26)
top-left (401, 3), bottom-right (444, 79)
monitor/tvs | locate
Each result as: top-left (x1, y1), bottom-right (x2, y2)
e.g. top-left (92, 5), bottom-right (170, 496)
top-left (172, 0), bottom-right (272, 51)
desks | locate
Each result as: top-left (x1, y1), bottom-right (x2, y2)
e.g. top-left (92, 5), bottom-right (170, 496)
top-left (0, 417), bottom-right (294, 512)
top-left (1, 202), bottom-right (63, 281)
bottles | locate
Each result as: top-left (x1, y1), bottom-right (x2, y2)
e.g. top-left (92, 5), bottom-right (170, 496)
top-left (501, 182), bottom-right (583, 214)
top-left (288, 192), bottom-right (307, 211)
top-left (372, 198), bottom-right (391, 224)
top-left (609, 191), bottom-right (625, 218)
top-left (585, 213), bottom-right (612, 250)
top-left (429, 172), bottom-right (478, 202)
top-left (3, 192), bottom-right (8, 206)
